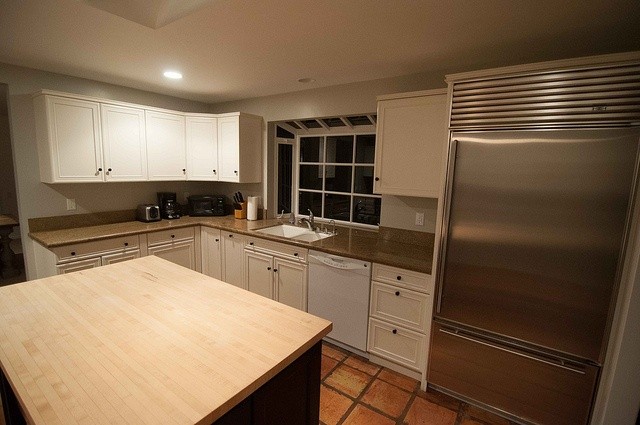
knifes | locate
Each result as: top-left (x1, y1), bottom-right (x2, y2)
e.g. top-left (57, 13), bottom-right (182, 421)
top-left (233, 192), bottom-right (243, 210)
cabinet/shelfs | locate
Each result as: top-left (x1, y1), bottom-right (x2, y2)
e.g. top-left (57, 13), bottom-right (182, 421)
top-left (368, 263), bottom-right (430, 373)
top-left (186, 115), bottom-right (216, 180)
top-left (144, 109), bottom-right (187, 180)
top-left (220, 235), bottom-right (244, 291)
top-left (55, 235), bottom-right (143, 273)
top-left (372, 94), bottom-right (452, 195)
top-left (146, 227), bottom-right (195, 275)
top-left (244, 233), bottom-right (309, 313)
top-left (216, 115), bottom-right (240, 182)
top-left (200, 227), bottom-right (220, 277)
top-left (49, 95), bottom-right (148, 183)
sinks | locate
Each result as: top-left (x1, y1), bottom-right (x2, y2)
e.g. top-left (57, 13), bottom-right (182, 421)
top-left (294, 232), bottom-right (328, 242)
top-left (261, 225), bottom-right (303, 237)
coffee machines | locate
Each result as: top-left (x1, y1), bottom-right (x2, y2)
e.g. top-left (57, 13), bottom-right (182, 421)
top-left (157, 192), bottom-right (181, 219)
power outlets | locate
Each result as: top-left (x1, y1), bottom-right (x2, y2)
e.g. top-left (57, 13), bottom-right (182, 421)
top-left (67, 198), bottom-right (77, 210)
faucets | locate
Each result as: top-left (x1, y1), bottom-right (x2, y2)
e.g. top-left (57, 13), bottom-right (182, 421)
top-left (323, 220), bottom-right (336, 234)
top-left (289, 213), bottom-right (295, 225)
top-left (299, 209), bottom-right (317, 231)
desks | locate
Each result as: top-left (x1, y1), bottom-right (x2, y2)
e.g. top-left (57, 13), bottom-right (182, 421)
top-left (0, 255), bottom-right (333, 425)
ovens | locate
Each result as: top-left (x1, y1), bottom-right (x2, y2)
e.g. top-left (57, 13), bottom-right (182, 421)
top-left (136, 204), bottom-right (161, 222)
top-left (188, 194), bottom-right (225, 216)
